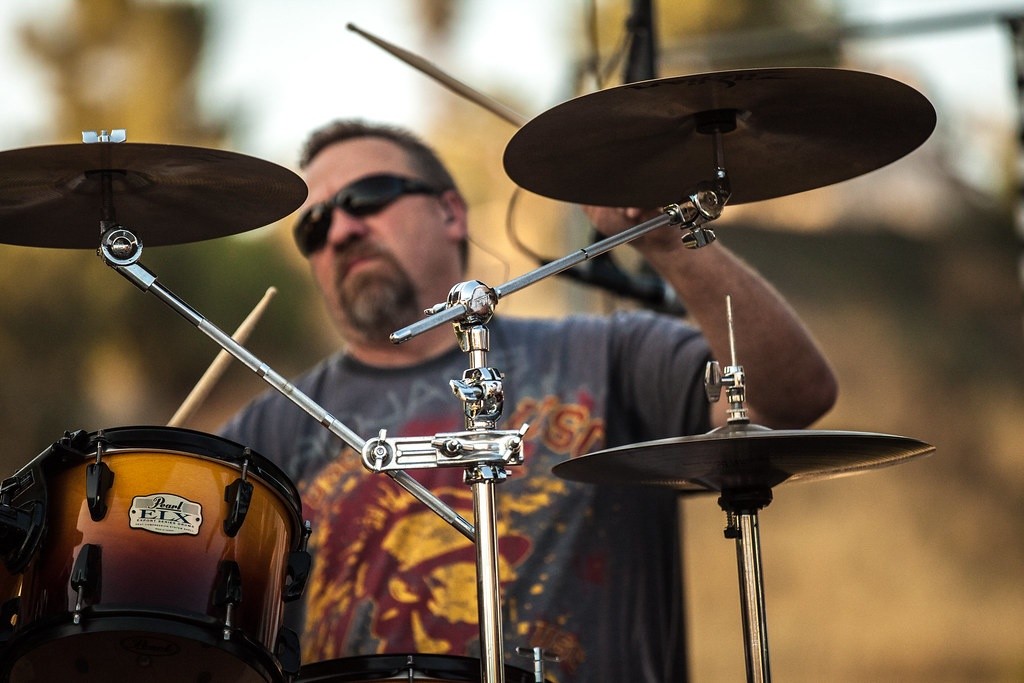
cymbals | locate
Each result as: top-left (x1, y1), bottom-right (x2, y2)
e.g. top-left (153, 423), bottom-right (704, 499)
top-left (551, 418), bottom-right (938, 496)
top-left (503, 67), bottom-right (938, 208)
top-left (0, 131), bottom-right (308, 249)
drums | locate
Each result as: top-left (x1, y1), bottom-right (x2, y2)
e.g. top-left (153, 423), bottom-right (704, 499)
top-left (0, 423), bottom-right (313, 683)
top-left (290, 649), bottom-right (550, 683)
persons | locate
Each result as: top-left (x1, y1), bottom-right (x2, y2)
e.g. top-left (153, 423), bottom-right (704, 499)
top-left (217, 122), bottom-right (838, 683)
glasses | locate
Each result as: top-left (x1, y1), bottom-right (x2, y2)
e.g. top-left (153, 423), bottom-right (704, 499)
top-left (291, 171), bottom-right (442, 259)
